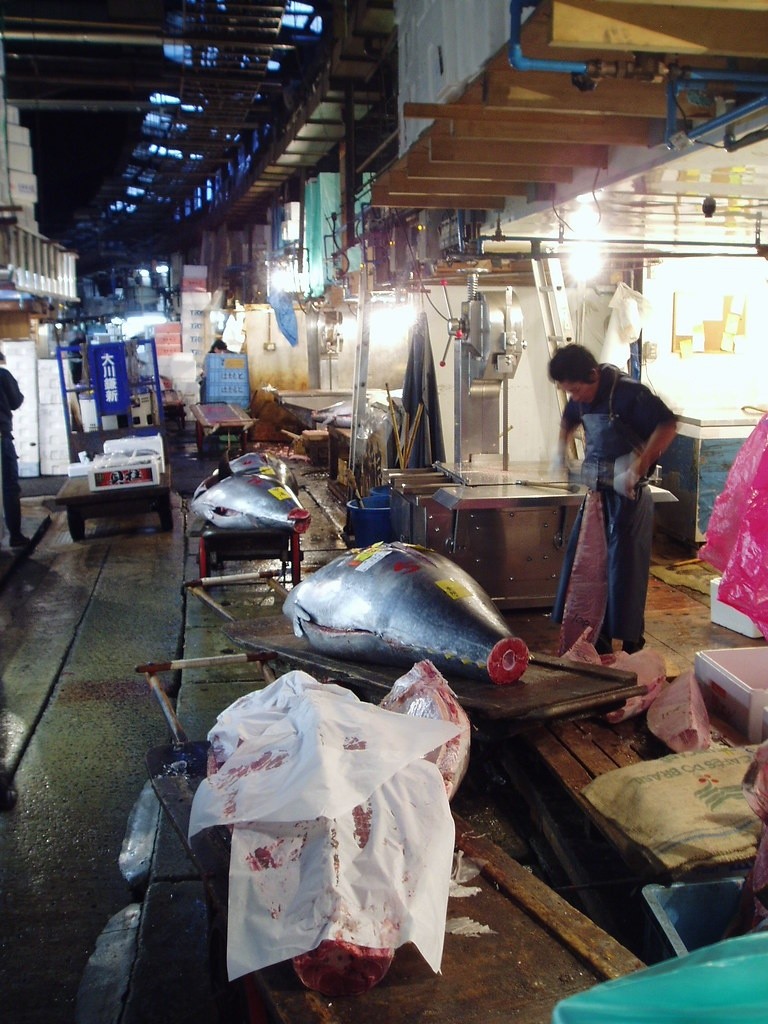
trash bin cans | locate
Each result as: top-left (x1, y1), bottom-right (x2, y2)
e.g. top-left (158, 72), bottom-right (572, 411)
top-left (345, 494), bottom-right (391, 549)
top-left (368, 484), bottom-right (391, 496)
top-left (639, 875), bottom-right (768, 968)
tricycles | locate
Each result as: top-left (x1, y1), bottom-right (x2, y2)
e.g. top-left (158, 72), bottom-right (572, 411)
top-left (54, 338), bottom-right (174, 541)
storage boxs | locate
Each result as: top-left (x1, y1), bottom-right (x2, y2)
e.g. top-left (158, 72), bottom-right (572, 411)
top-left (693, 644), bottom-right (767, 736)
top-left (639, 875), bottom-right (760, 963)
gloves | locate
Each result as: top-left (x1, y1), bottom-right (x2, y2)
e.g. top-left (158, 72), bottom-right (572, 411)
top-left (547, 452), bottom-right (570, 478)
top-left (613, 468), bottom-right (640, 501)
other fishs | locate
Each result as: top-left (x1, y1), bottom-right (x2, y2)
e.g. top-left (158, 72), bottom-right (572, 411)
top-left (282, 540), bottom-right (529, 686)
top-left (188, 451), bottom-right (312, 534)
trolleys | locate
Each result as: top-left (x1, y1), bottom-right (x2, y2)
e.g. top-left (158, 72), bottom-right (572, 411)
top-left (130, 651), bottom-right (652, 1024)
top-left (188, 399), bottom-right (252, 457)
top-left (199, 518), bottom-right (302, 589)
top-left (184, 570), bottom-right (636, 803)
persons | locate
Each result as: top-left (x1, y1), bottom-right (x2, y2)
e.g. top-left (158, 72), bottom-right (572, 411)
top-left (547, 342), bottom-right (678, 658)
top-left (0, 351), bottom-right (31, 548)
top-left (200, 340), bottom-right (227, 404)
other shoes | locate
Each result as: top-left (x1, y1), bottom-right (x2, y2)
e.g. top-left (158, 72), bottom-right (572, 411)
top-left (622, 634), bottom-right (646, 655)
top-left (10, 532), bottom-right (31, 547)
top-left (595, 637), bottom-right (613, 655)
